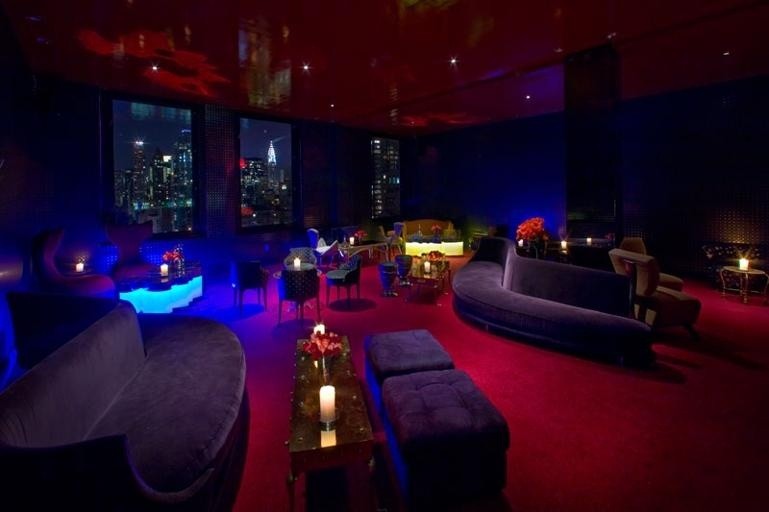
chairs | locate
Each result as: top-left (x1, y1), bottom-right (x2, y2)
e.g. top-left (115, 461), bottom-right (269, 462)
top-left (102, 219), bottom-right (153, 282)
top-left (618, 235), bottom-right (683, 293)
top-left (229, 216), bottom-right (466, 327)
top-left (32, 225), bottom-right (115, 295)
top-left (607, 247), bottom-right (700, 341)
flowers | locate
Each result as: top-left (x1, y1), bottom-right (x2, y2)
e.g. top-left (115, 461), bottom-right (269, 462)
top-left (303, 330), bottom-right (345, 363)
top-left (515, 217), bottom-right (546, 243)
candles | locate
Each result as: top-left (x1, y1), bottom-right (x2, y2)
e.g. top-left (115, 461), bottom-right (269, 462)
top-left (318, 384), bottom-right (336, 423)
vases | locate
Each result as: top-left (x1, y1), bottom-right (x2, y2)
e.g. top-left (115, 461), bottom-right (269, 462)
top-left (318, 357), bottom-right (330, 386)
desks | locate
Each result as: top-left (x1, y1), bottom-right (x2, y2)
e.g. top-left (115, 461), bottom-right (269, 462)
top-left (718, 265), bottom-right (769, 304)
top-left (115, 268), bottom-right (204, 314)
top-left (287, 334), bottom-right (382, 512)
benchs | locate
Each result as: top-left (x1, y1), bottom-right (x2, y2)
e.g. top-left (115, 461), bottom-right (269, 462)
top-left (0, 290), bottom-right (247, 512)
top-left (451, 236), bottom-right (658, 374)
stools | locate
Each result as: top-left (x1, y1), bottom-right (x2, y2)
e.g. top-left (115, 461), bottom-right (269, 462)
top-left (364, 328), bottom-right (456, 411)
top-left (381, 367), bottom-right (511, 510)
